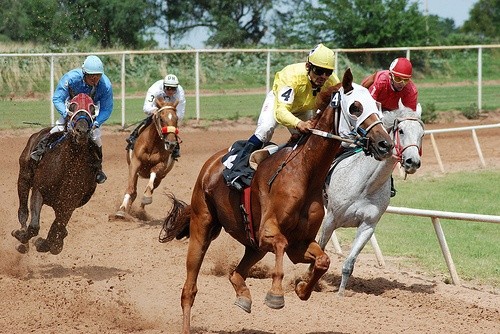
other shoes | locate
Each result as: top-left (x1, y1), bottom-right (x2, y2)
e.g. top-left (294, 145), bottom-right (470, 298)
top-left (30, 147), bottom-right (43, 162)
top-left (125, 133), bottom-right (137, 144)
top-left (96, 171), bottom-right (108, 184)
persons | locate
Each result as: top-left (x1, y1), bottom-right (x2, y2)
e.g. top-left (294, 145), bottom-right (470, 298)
top-left (222, 43), bottom-right (340, 191)
top-left (30, 56), bottom-right (113, 184)
top-left (360, 57), bottom-right (418, 112)
top-left (126, 74), bottom-right (186, 144)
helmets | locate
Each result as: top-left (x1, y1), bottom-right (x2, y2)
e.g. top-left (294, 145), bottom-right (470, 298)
top-left (163, 74), bottom-right (178, 86)
top-left (307, 43), bottom-right (336, 70)
top-left (389, 57), bottom-right (412, 78)
top-left (82, 55), bottom-right (105, 75)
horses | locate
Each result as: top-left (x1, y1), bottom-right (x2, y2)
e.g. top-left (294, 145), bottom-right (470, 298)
top-left (115, 97), bottom-right (180, 220)
top-left (11, 83), bottom-right (102, 255)
top-left (158, 68), bottom-right (395, 334)
top-left (316, 98), bottom-right (425, 297)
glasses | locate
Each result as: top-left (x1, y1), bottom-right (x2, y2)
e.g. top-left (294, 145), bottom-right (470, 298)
top-left (392, 74), bottom-right (410, 84)
top-left (312, 67), bottom-right (333, 76)
top-left (165, 86), bottom-right (177, 91)
